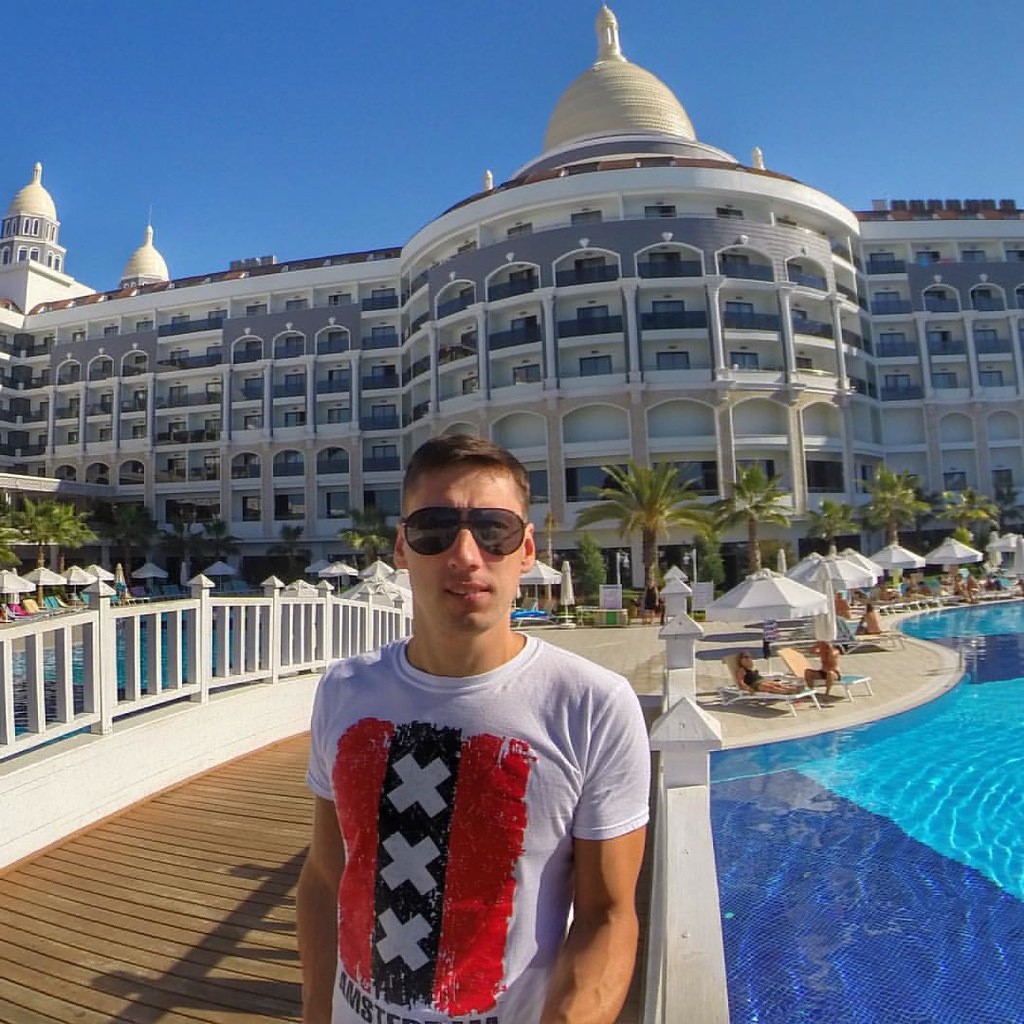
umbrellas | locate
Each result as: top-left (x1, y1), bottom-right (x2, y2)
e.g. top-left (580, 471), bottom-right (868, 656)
top-left (706, 533), bottom-right (1023, 671)
top-left (0, 562), bottom-right (187, 621)
top-left (203, 561), bottom-right (239, 593)
top-left (515, 560), bottom-right (577, 622)
top-left (304, 559), bottom-right (411, 613)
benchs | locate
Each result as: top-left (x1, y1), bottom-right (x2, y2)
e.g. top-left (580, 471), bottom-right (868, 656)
top-left (637, 609), bottom-right (654, 624)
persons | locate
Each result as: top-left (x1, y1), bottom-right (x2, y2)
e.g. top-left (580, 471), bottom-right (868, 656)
top-left (641, 578), bottom-right (659, 625)
top-left (735, 560), bottom-right (1016, 699)
top-left (295, 433), bottom-right (651, 1024)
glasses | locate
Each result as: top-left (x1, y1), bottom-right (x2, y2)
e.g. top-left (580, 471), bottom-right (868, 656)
top-left (402, 505), bottom-right (527, 556)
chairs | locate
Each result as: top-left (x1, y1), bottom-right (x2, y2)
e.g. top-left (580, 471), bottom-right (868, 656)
top-left (768, 578), bottom-right (1016, 656)
top-left (717, 648), bottom-right (875, 717)
top-left (510, 597), bottom-right (557, 628)
top-left (0, 581), bottom-right (249, 622)
